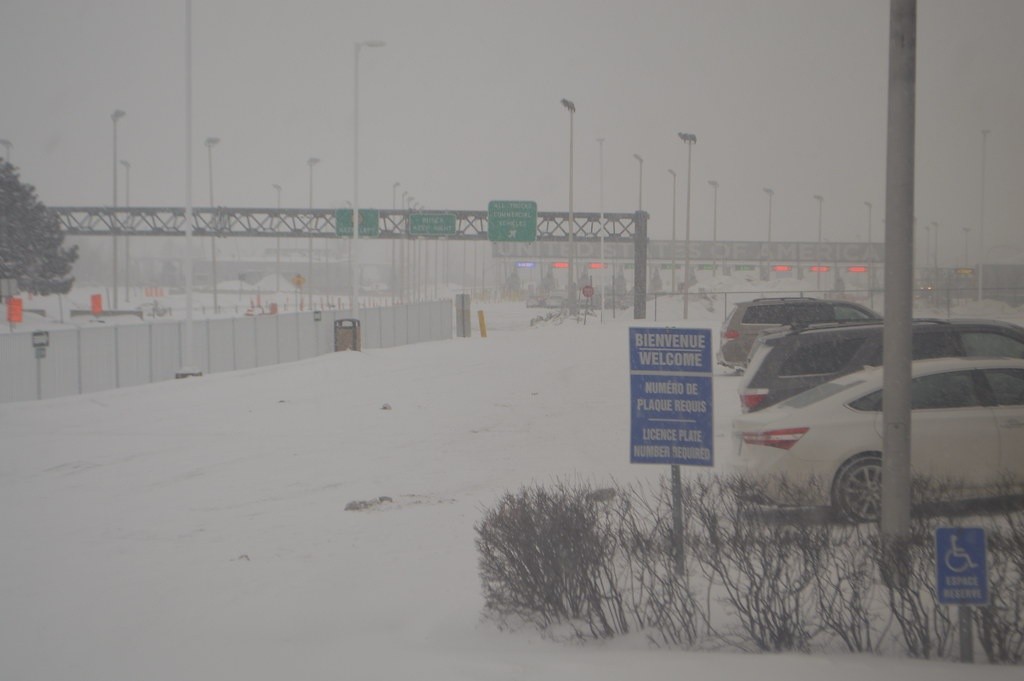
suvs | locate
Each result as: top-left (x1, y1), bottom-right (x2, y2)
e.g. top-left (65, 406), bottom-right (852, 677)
top-left (741, 361), bottom-right (1024, 523)
top-left (736, 317), bottom-right (1024, 412)
top-left (715, 296), bottom-right (884, 374)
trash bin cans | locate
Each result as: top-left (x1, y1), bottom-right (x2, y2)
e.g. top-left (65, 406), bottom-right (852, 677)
top-left (334, 319), bottom-right (361, 351)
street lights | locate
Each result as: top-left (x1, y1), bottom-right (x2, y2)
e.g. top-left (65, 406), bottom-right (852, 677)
top-left (203, 135), bottom-right (227, 315)
top-left (632, 153), bottom-right (643, 209)
top-left (108, 106), bottom-right (132, 309)
top-left (813, 195), bottom-right (822, 244)
top-left (667, 168), bottom-right (676, 240)
top-left (863, 201), bottom-right (969, 308)
top-left (349, 40), bottom-right (388, 308)
top-left (679, 132), bottom-right (691, 319)
top-left (763, 188), bottom-right (773, 242)
top-left (560, 97), bottom-right (578, 320)
top-left (595, 133), bottom-right (608, 321)
top-left (391, 181), bottom-right (453, 305)
top-left (708, 179), bottom-right (717, 243)
top-left (979, 129), bottom-right (990, 309)
top-left (273, 183), bottom-right (282, 303)
top-left (305, 157), bottom-right (320, 311)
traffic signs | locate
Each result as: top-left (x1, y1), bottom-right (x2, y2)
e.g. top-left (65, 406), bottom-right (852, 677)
top-left (487, 200), bottom-right (538, 243)
